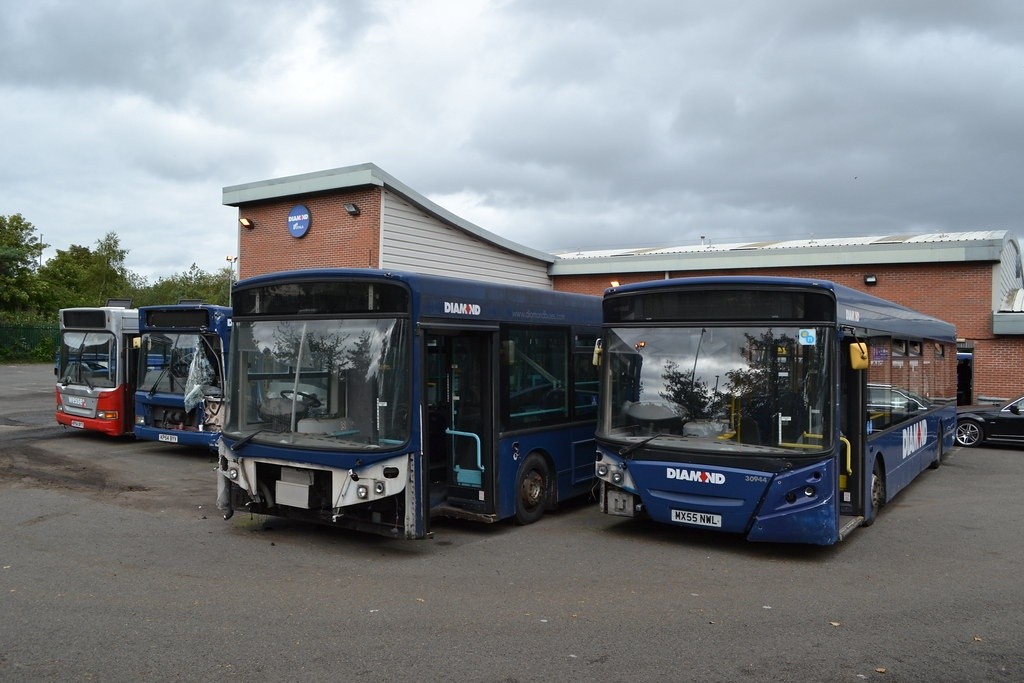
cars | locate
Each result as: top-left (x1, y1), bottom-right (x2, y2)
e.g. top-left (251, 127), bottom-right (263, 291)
top-left (866, 382), bottom-right (934, 412)
top-left (955, 394), bottom-right (1024, 450)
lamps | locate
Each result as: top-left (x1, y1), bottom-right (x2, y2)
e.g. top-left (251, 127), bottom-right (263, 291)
top-left (343, 203), bottom-right (359, 215)
top-left (610, 281), bottom-right (620, 287)
top-left (864, 274), bottom-right (877, 285)
top-left (239, 218), bottom-right (254, 229)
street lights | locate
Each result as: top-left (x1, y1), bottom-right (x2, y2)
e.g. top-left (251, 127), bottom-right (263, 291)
top-left (226, 255), bottom-right (237, 306)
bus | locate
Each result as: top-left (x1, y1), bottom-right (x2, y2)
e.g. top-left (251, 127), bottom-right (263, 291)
top-left (594, 276), bottom-right (958, 545)
top-left (52, 307), bottom-right (140, 439)
top-left (215, 267), bottom-right (602, 543)
top-left (133, 299), bottom-right (233, 450)
